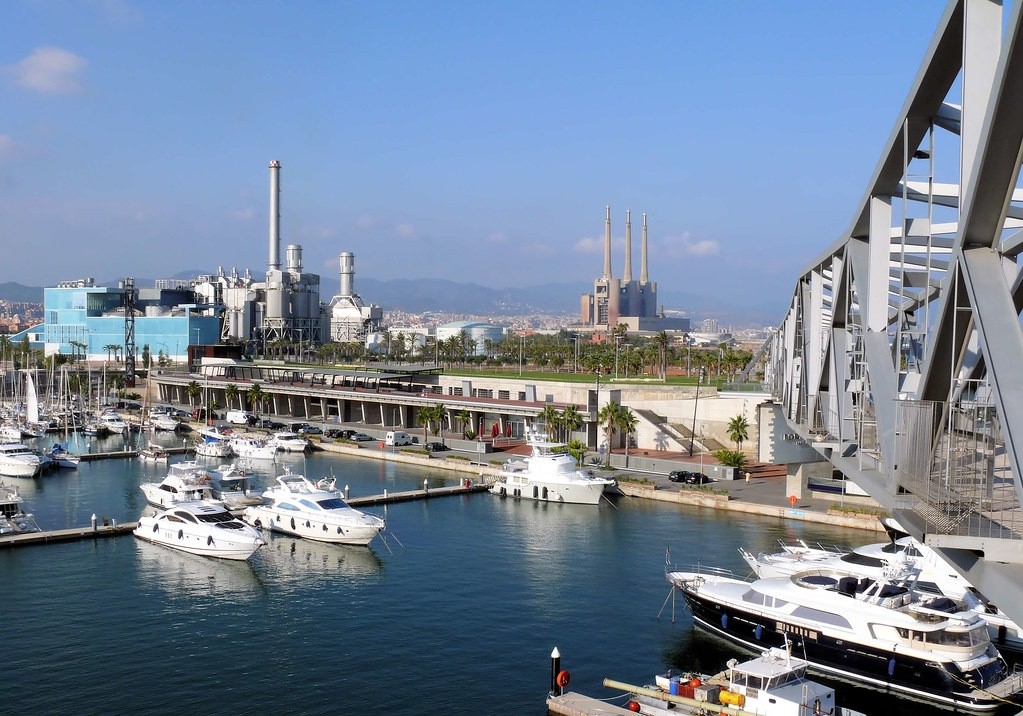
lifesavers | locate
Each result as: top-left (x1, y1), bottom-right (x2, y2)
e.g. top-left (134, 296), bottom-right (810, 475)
top-left (789, 495), bottom-right (797, 504)
top-left (239, 470), bottom-right (245, 476)
top-left (557, 670), bottom-right (570, 688)
top-left (210, 420), bottom-right (213, 424)
top-left (379, 443), bottom-right (384, 448)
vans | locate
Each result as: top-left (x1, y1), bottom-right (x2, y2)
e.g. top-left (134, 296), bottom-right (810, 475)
top-left (385, 430), bottom-right (412, 447)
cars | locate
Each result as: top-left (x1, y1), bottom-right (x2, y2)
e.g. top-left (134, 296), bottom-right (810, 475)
top-left (424, 442), bottom-right (448, 452)
top-left (668, 470), bottom-right (691, 482)
top-left (684, 472), bottom-right (708, 484)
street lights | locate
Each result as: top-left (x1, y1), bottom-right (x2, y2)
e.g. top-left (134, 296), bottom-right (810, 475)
top-left (613, 333), bottom-right (634, 379)
top-left (569, 334), bottom-right (580, 374)
top-left (686, 339), bottom-right (691, 378)
top-left (519, 336), bottom-right (525, 377)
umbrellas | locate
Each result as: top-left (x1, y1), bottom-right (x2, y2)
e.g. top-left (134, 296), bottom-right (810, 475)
top-left (479, 423), bottom-right (483, 442)
top-left (492, 424), bottom-right (496, 447)
top-left (495, 422), bottom-right (499, 445)
top-left (506, 423), bottom-right (512, 444)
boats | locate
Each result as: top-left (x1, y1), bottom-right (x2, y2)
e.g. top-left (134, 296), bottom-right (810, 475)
top-left (132, 484), bottom-right (267, 560)
top-left (241, 461), bottom-right (386, 547)
top-left (656, 516), bottom-right (1023, 716)
top-left (486, 419), bottom-right (616, 506)
top-left (0, 347), bottom-right (375, 509)
top-left (546, 630), bottom-right (869, 716)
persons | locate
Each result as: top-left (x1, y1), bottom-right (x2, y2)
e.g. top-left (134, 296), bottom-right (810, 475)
top-left (744, 471), bottom-right (751, 486)
